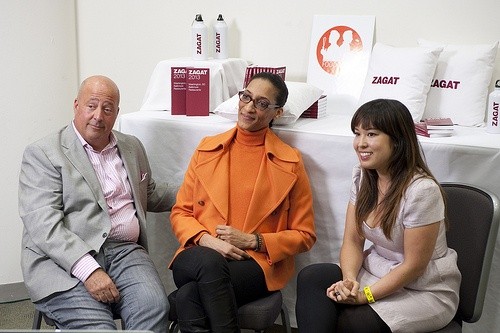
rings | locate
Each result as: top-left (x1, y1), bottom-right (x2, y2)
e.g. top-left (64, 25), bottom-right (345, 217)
top-left (335, 292), bottom-right (340, 295)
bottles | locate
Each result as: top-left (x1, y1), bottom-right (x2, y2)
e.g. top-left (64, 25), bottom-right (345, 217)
top-left (191, 14), bottom-right (208, 61)
top-left (213, 14), bottom-right (228, 59)
top-left (487, 80), bottom-right (500, 134)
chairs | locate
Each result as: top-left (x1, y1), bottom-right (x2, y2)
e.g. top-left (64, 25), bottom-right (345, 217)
top-left (166, 289), bottom-right (292, 333)
top-left (433, 181), bottom-right (500, 333)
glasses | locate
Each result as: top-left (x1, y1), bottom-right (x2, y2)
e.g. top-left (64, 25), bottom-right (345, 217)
top-left (238, 90), bottom-right (281, 111)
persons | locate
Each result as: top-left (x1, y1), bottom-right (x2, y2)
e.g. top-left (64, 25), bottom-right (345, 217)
top-left (168, 72), bottom-right (318, 333)
top-left (19, 75), bottom-right (179, 333)
top-left (297, 100), bottom-right (463, 333)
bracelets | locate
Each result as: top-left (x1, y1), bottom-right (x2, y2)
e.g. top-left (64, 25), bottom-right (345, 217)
top-left (363, 286), bottom-right (375, 303)
top-left (255, 232), bottom-right (261, 252)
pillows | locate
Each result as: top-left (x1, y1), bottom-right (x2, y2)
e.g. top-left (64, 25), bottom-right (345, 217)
top-left (356, 42), bottom-right (443, 125)
top-left (212, 81), bottom-right (324, 125)
top-left (418, 38), bottom-right (500, 126)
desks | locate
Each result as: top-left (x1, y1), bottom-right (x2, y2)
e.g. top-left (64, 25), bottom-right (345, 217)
top-left (115, 111), bottom-right (500, 333)
